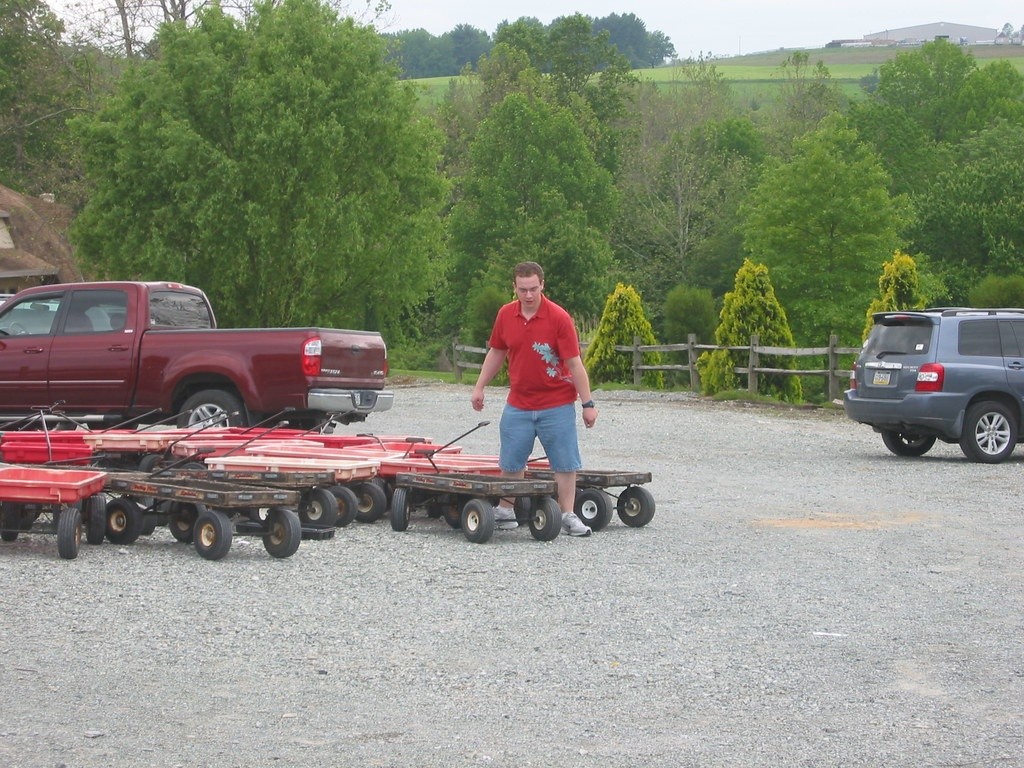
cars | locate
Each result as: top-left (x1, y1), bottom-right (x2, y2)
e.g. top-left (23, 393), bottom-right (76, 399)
top-left (0, 292), bottom-right (133, 342)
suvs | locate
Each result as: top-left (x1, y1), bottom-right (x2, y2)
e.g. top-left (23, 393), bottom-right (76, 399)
top-left (843, 306), bottom-right (1024, 465)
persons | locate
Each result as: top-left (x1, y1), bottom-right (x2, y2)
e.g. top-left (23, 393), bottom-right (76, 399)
top-left (470, 261), bottom-right (597, 537)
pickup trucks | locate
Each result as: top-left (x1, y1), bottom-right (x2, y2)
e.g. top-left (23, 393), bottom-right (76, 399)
top-left (0, 280), bottom-right (395, 439)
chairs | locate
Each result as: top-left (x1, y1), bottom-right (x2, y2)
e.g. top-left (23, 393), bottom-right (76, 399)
top-left (64, 308), bottom-right (92, 332)
top-left (110, 314), bottom-right (125, 331)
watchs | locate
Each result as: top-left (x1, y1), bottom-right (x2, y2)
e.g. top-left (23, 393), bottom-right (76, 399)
top-left (582, 400), bottom-right (595, 408)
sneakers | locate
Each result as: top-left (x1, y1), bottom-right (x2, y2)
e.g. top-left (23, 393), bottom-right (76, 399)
top-left (561, 512), bottom-right (592, 536)
top-left (491, 507), bottom-right (518, 529)
